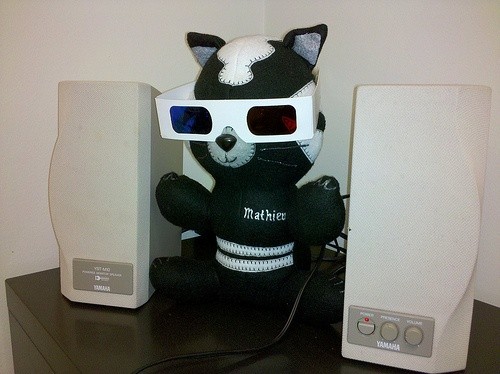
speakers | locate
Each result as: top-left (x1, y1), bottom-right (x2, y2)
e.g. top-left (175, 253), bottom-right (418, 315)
top-left (341, 85), bottom-right (491, 374)
top-left (48, 81), bottom-right (184, 309)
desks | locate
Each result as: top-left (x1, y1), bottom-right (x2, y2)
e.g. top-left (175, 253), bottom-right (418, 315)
top-left (5, 235), bottom-right (500, 374)
